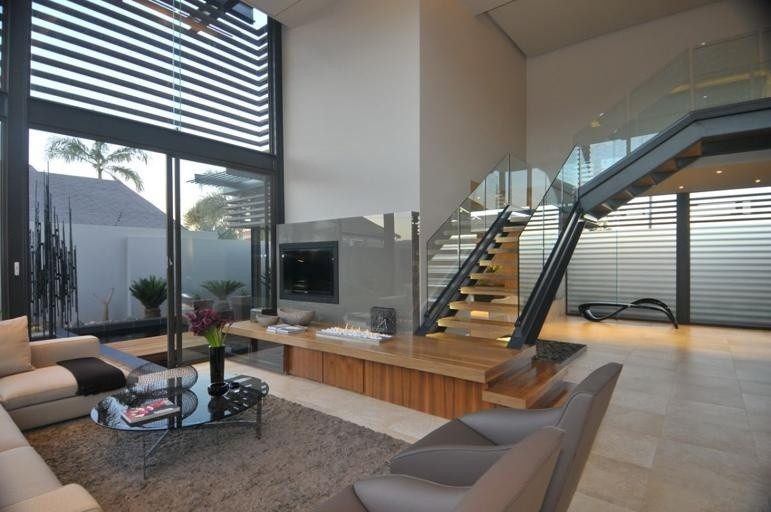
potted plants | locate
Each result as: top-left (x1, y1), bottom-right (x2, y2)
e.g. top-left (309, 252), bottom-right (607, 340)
top-left (232, 287), bottom-right (251, 308)
top-left (202, 280), bottom-right (243, 309)
top-left (129, 275), bottom-right (169, 318)
top-left (191, 291), bottom-right (207, 310)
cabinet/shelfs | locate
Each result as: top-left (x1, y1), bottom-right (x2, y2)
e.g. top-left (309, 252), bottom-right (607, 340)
top-left (286, 348), bottom-right (366, 393)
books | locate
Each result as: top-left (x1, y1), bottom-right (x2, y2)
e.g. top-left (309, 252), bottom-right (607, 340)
top-left (118, 398), bottom-right (181, 424)
top-left (266, 323), bottom-right (309, 335)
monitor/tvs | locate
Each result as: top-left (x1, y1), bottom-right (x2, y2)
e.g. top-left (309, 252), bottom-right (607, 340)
top-left (278, 241), bottom-right (338, 305)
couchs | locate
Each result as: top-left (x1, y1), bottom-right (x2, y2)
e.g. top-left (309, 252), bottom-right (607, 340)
top-left (393, 360), bottom-right (624, 512)
top-left (0, 316), bottom-right (134, 512)
top-left (313, 427), bottom-right (565, 511)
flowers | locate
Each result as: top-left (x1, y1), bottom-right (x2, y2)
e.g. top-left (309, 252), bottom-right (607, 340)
top-left (187, 308), bottom-right (234, 346)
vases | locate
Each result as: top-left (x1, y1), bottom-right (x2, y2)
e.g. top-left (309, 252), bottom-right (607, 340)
top-left (210, 344), bottom-right (226, 384)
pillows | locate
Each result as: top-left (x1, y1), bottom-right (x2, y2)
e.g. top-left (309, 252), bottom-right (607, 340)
top-left (0, 316), bottom-right (34, 380)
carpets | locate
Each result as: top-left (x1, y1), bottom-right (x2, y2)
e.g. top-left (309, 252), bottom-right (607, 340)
top-left (21, 381), bottom-right (413, 512)
